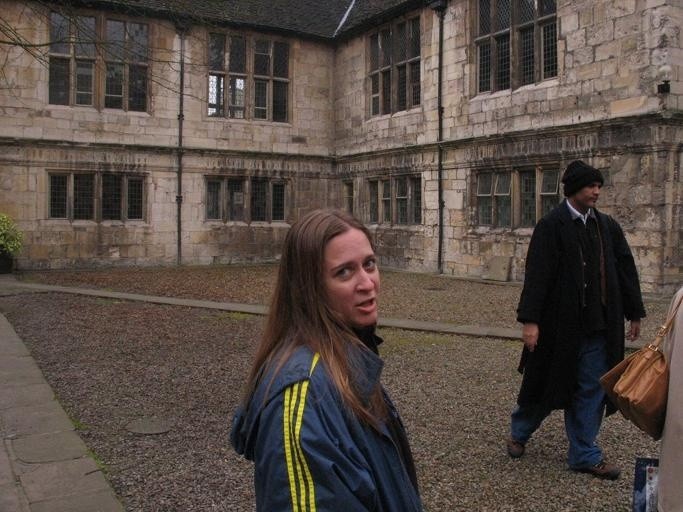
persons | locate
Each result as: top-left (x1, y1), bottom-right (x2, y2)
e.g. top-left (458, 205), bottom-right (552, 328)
top-left (656, 281), bottom-right (683, 511)
top-left (229, 209), bottom-right (421, 510)
top-left (504, 159), bottom-right (643, 480)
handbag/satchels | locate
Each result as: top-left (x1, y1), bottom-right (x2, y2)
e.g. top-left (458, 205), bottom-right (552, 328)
top-left (600, 337), bottom-right (670, 441)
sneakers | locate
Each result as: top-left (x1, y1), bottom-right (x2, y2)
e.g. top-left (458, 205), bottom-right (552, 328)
top-left (570, 453), bottom-right (622, 480)
top-left (508, 433), bottom-right (524, 459)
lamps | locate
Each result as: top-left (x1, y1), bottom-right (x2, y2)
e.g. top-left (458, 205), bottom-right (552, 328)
top-left (658, 66), bottom-right (673, 92)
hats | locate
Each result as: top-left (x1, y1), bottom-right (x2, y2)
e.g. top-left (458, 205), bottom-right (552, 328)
top-left (562, 161), bottom-right (603, 197)
